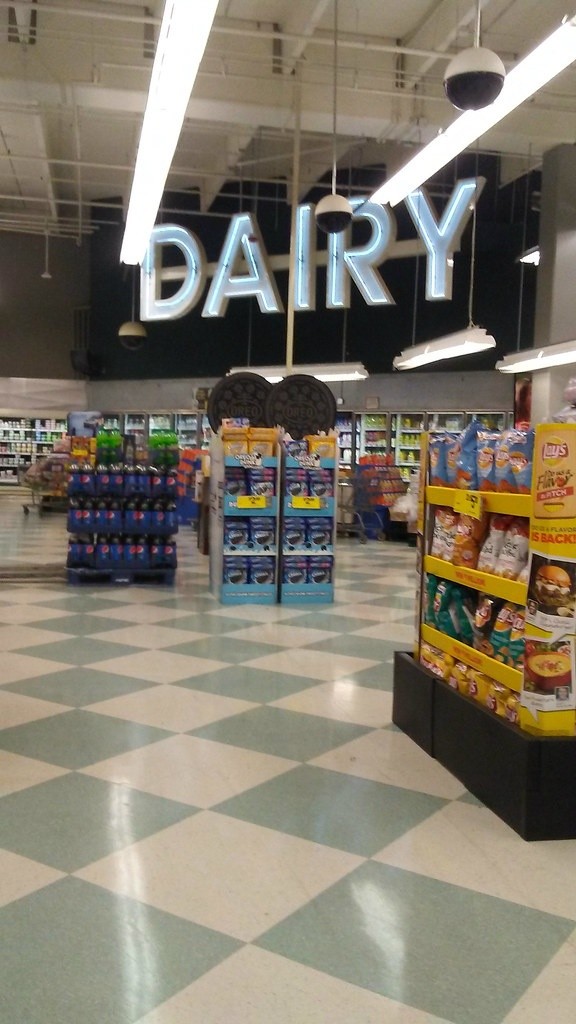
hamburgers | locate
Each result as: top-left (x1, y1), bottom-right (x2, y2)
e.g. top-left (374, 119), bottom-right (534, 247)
top-left (535, 565), bottom-right (571, 598)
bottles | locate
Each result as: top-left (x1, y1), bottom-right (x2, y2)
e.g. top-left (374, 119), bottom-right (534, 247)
top-left (68, 418), bottom-right (181, 568)
top-left (359, 451), bottom-right (407, 506)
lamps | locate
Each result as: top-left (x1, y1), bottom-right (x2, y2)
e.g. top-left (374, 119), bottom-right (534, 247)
top-left (225, 134), bottom-right (370, 384)
top-left (368, 13), bottom-right (576, 207)
top-left (494, 142), bottom-right (576, 374)
top-left (392, 115), bottom-right (497, 372)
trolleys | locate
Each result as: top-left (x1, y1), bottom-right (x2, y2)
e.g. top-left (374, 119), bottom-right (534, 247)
top-left (336, 464), bottom-right (395, 545)
top-left (16, 456), bottom-right (71, 515)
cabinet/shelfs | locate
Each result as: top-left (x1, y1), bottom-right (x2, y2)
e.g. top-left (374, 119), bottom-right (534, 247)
top-left (412, 423), bottom-right (575, 734)
top-left (0, 410), bottom-right (514, 604)
